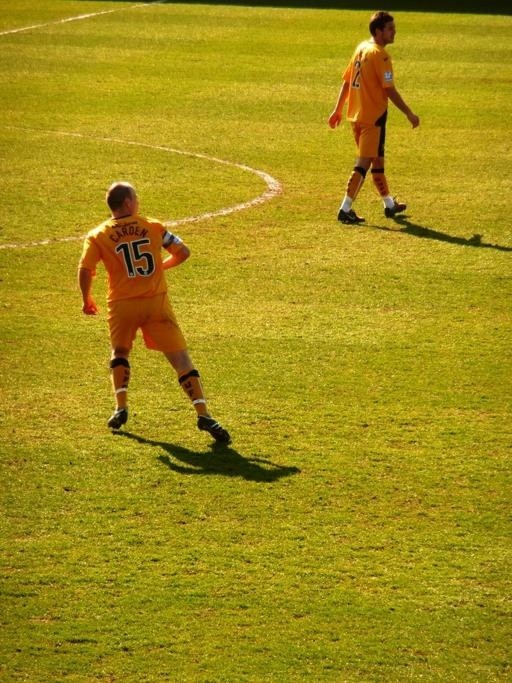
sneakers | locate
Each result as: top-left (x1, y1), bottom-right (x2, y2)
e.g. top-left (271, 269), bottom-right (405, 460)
top-left (107, 408), bottom-right (127, 428)
top-left (198, 416), bottom-right (230, 441)
top-left (385, 202), bottom-right (406, 215)
top-left (338, 208), bottom-right (365, 221)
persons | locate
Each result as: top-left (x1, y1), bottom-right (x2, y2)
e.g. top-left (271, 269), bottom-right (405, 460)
top-left (77, 179), bottom-right (231, 443)
top-left (328, 9), bottom-right (420, 225)
